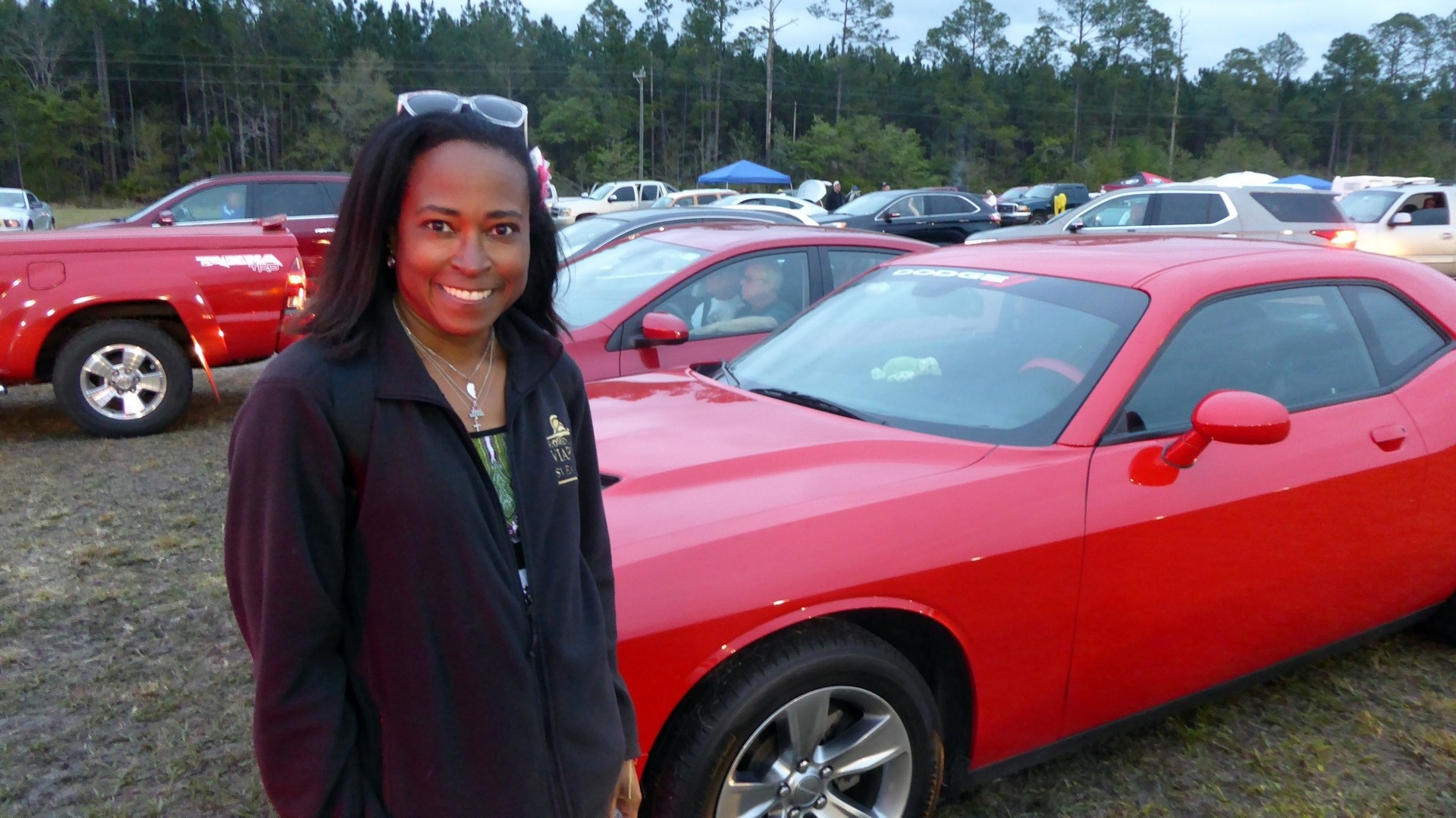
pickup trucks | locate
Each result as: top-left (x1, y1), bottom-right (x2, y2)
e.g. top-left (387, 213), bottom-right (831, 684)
top-left (0, 213), bottom-right (313, 440)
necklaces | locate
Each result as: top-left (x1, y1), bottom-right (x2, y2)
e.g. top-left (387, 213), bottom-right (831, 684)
top-left (393, 299), bottom-right (496, 431)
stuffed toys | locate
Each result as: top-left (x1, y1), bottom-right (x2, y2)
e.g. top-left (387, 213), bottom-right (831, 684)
top-left (871, 356), bottom-right (941, 382)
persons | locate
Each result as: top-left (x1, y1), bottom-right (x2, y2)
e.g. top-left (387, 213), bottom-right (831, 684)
top-left (844, 185), bottom-right (862, 204)
top-left (733, 260), bottom-right (801, 327)
top-left (691, 266), bottom-right (746, 329)
top-left (825, 180), bottom-right (843, 211)
top-left (221, 89), bottom-right (642, 818)
top-left (881, 182), bottom-right (890, 191)
top-left (1422, 198), bottom-right (1437, 209)
top-left (222, 187), bottom-right (244, 219)
top-left (982, 190), bottom-right (997, 210)
top-left (1130, 202), bottom-right (1147, 225)
top-left (777, 189), bottom-right (786, 196)
top-left (908, 197), bottom-right (920, 216)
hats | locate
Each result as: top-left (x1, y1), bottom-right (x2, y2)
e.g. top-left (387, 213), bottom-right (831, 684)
top-left (881, 182), bottom-right (889, 188)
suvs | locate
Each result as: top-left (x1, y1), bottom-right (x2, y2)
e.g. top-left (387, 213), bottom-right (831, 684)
top-left (61, 172), bottom-right (351, 300)
top-left (965, 184), bottom-right (1357, 247)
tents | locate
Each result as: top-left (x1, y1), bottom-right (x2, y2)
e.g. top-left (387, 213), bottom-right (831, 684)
top-left (1100, 172), bottom-right (1175, 191)
top-left (1200, 171), bottom-right (1279, 188)
top-left (696, 159), bottom-right (793, 196)
top-left (1269, 174), bottom-right (1332, 190)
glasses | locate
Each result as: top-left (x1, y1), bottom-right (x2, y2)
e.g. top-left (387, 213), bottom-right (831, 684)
top-left (396, 89), bottom-right (529, 155)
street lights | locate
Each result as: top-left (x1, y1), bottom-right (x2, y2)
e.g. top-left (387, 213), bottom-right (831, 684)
top-left (632, 66), bottom-right (645, 181)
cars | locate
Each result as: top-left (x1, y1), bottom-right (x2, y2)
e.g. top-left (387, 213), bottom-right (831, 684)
top-left (580, 236), bottom-right (1456, 818)
top-left (811, 175), bottom-right (1101, 248)
top-left (0, 187), bottom-right (57, 235)
top-left (545, 180), bottom-right (942, 385)
top-left (1333, 178), bottom-right (1455, 277)
